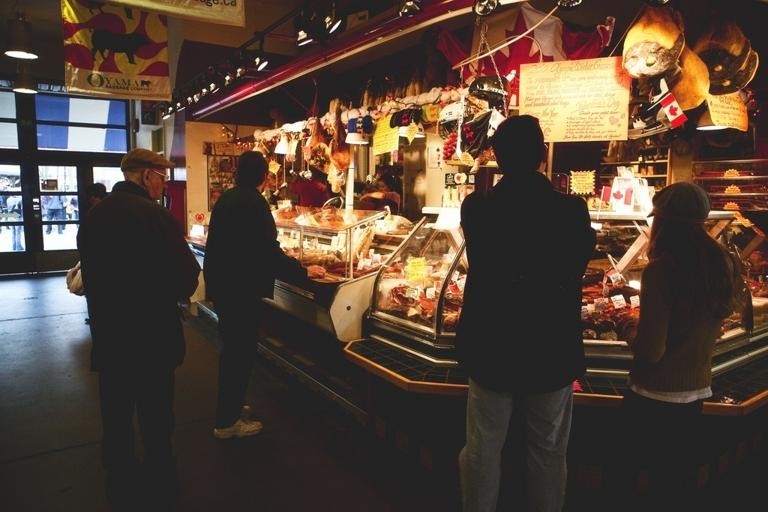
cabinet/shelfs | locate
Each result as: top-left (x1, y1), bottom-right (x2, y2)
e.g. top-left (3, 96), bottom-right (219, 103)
top-left (188, 202), bottom-right (765, 509)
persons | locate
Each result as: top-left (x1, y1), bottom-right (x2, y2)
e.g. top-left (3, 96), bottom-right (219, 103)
top-left (614, 181), bottom-right (739, 512)
top-left (359, 174), bottom-right (401, 215)
top-left (87, 183), bottom-right (108, 205)
top-left (456, 114), bottom-right (596, 512)
top-left (76, 147), bottom-right (201, 468)
top-left (0, 176), bottom-right (78, 250)
top-left (291, 169), bottom-right (335, 207)
top-left (205, 151), bottom-right (327, 438)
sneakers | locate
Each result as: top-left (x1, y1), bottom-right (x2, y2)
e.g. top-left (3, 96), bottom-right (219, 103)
top-left (213, 404), bottom-right (263, 440)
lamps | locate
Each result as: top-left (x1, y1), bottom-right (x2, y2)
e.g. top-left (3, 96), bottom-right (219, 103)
top-left (159, 0), bottom-right (499, 121)
top-left (2, 1), bottom-right (41, 95)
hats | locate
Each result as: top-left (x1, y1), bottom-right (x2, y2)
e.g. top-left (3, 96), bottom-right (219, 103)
top-left (646, 181), bottom-right (710, 224)
top-left (120, 147), bottom-right (177, 171)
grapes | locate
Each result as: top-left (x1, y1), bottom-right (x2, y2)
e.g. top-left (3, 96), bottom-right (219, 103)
top-left (442, 132), bottom-right (457, 160)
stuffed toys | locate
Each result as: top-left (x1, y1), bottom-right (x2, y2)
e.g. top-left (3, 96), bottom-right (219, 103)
top-left (620, 2), bottom-right (760, 138)
top-left (460, 74), bottom-right (512, 175)
